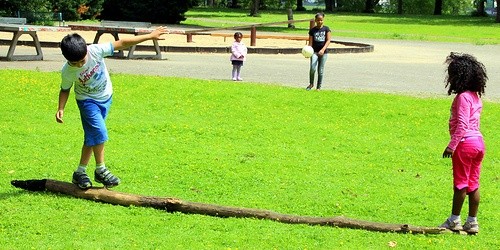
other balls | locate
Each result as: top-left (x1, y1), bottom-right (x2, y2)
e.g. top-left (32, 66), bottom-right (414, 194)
top-left (301, 46), bottom-right (314, 58)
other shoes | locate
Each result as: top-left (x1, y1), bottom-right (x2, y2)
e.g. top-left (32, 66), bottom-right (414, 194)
top-left (232, 78), bottom-right (236, 81)
top-left (237, 78), bottom-right (243, 81)
top-left (316, 88), bottom-right (320, 91)
top-left (306, 85), bottom-right (314, 91)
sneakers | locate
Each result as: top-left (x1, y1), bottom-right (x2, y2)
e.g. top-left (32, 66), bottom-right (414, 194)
top-left (72, 171), bottom-right (92, 190)
top-left (461, 219), bottom-right (479, 234)
top-left (94, 168), bottom-right (122, 187)
top-left (440, 217), bottom-right (464, 231)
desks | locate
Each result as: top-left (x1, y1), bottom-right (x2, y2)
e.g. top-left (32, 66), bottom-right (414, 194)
top-left (0, 17), bottom-right (71, 61)
top-left (68, 19), bottom-right (185, 60)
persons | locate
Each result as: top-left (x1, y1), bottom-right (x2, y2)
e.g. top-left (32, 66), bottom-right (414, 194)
top-left (57, 26), bottom-right (169, 189)
top-left (306, 13), bottom-right (331, 91)
top-left (230, 32), bottom-right (247, 80)
top-left (442, 51), bottom-right (489, 232)
